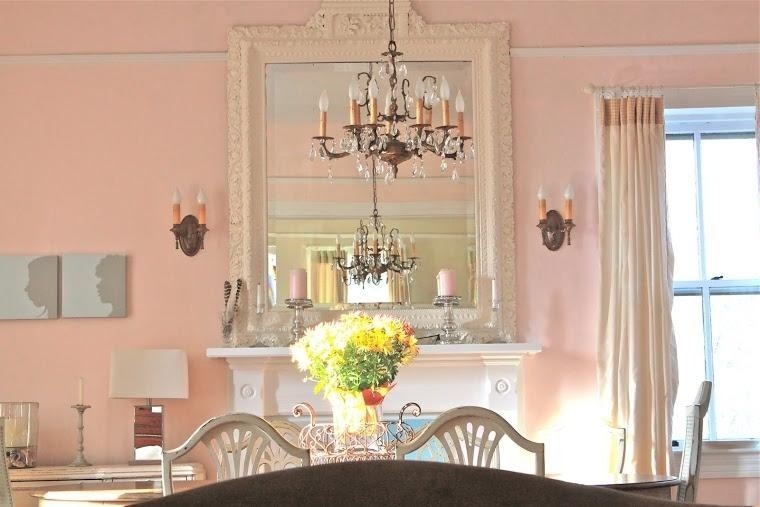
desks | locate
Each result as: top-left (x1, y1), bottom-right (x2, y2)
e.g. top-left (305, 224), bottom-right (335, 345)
top-left (8, 465), bottom-right (208, 490)
top-left (26, 472), bottom-right (682, 506)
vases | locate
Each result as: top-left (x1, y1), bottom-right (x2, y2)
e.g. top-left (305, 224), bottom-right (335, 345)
top-left (330, 392), bottom-right (381, 445)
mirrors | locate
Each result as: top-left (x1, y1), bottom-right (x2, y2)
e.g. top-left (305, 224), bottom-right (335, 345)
top-left (225, 0), bottom-right (518, 350)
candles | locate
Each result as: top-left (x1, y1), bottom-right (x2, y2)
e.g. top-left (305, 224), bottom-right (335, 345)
top-left (256, 281), bottom-right (261, 311)
top-left (78, 377), bottom-right (84, 405)
top-left (492, 278), bottom-right (497, 306)
top-left (286, 269), bottom-right (307, 299)
top-left (438, 268), bottom-right (457, 296)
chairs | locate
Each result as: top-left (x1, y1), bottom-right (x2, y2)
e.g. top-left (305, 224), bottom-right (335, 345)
top-left (407, 423), bottom-right (502, 469)
top-left (216, 420), bottom-right (333, 479)
top-left (162, 412), bottom-right (312, 496)
top-left (676, 381), bottom-right (713, 501)
top-left (536, 421), bottom-right (625, 475)
top-left (393, 405), bottom-right (545, 478)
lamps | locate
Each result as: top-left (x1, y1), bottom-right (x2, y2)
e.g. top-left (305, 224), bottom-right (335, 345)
top-left (333, 155), bottom-right (421, 289)
top-left (536, 184), bottom-right (575, 251)
top-left (169, 187), bottom-right (209, 256)
top-left (108, 350), bottom-right (188, 464)
top-left (308, 0), bottom-right (478, 187)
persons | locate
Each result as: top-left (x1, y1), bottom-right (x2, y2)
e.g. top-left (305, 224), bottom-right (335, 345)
top-left (25, 257), bottom-right (56, 318)
top-left (95, 254), bottom-right (125, 317)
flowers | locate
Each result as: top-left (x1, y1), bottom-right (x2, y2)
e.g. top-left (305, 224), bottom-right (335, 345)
top-left (290, 314), bottom-right (422, 427)
